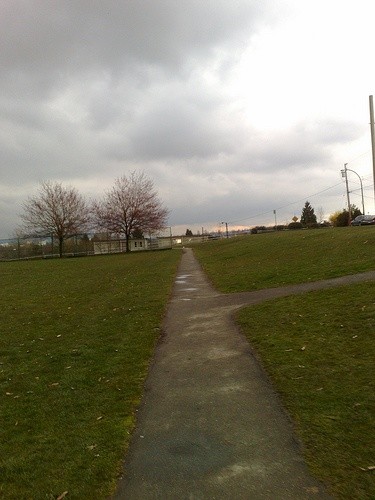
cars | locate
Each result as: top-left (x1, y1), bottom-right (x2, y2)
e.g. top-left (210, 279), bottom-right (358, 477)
top-left (350, 215), bottom-right (374, 226)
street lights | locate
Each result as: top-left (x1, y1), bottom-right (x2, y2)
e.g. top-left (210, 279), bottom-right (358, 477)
top-left (340, 169), bottom-right (365, 215)
